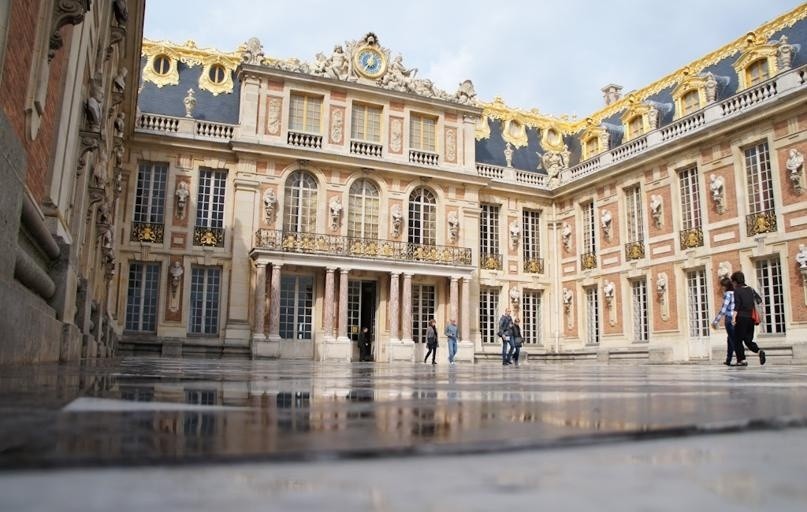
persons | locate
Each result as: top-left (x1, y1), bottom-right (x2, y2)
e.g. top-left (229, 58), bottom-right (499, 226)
top-left (708, 173), bottom-right (723, 194)
top-left (262, 186), bottom-right (278, 205)
top-left (711, 278), bottom-right (739, 366)
top-left (655, 272), bottom-right (668, 291)
top-left (444, 317), bottom-right (460, 367)
top-left (510, 287), bottom-right (522, 300)
top-left (387, 51), bottom-right (419, 89)
top-left (730, 271), bottom-right (767, 366)
top-left (357, 326), bottom-right (370, 363)
top-left (600, 208), bottom-right (612, 228)
top-left (87, 85), bottom-right (104, 125)
top-left (648, 193), bottom-right (662, 215)
top-left (314, 43), bottom-right (358, 81)
top-left (422, 319), bottom-right (440, 365)
top-left (496, 307), bottom-right (515, 365)
top-left (557, 221), bottom-right (572, 238)
top-left (447, 211), bottom-right (458, 225)
top-left (512, 317), bottom-right (522, 364)
top-left (170, 259), bottom-right (184, 281)
top-left (716, 262), bottom-right (730, 277)
top-left (93, 153), bottom-right (110, 189)
top-left (113, 109), bottom-right (128, 132)
top-left (602, 279), bottom-right (615, 297)
top-left (328, 195), bottom-right (343, 212)
top-left (391, 204), bottom-right (403, 219)
top-left (510, 220), bottom-right (522, 234)
top-left (173, 180), bottom-right (191, 200)
top-left (795, 243), bottom-right (807, 265)
top-left (560, 286), bottom-right (572, 304)
top-left (113, 64), bottom-right (129, 91)
top-left (785, 145), bottom-right (806, 171)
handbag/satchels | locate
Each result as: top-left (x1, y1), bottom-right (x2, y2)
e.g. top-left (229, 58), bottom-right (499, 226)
top-left (751, 303), bottom-right (760, 325)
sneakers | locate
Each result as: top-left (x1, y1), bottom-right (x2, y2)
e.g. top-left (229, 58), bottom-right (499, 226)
top-left (722, 350), bottom-right (765, 366)
top-left (422, 359), bottom-right (520, 369)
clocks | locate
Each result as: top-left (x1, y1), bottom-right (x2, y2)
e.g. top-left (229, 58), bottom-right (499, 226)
top-left (355, 46), bottom-right (387, 78)
